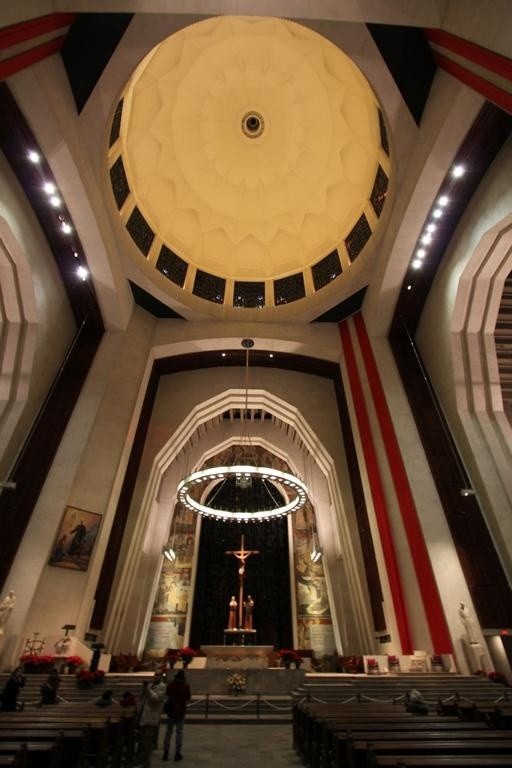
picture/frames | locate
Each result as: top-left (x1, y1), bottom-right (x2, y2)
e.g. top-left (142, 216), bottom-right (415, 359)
top-left (49, 505), bottom-right (103, 573)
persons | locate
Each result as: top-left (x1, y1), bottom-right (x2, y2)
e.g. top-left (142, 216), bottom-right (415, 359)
top-left (227, 594), bottom-right (237, 629)
top-left (457, 599), bottom-right (478, 644)
top-left (243, 594), bottom-right (256, 630)
top-left (89, 670), bottom-right (192, 761)
top-left (53, 516), bottom-right (88, 564)
top-left (0, 666), bottom-right (67, 711)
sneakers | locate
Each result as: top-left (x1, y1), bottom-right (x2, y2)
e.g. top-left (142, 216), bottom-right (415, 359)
top-left (161, 750), bottom-right (169, 760)
top-left (173, 750), bottom-right (183, 761)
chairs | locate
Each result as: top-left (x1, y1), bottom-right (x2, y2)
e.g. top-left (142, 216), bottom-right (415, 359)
top-left (365, 655), bottom-right (400, 674)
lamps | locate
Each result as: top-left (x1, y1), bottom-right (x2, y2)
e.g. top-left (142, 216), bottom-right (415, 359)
top-left (176, 337), bottom-right (309, 526)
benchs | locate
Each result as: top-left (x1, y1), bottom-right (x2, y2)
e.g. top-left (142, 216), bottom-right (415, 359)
top-left (292, 696), bottom-right (509, 768)
top-left (1, 699), bottom-right (142, 767)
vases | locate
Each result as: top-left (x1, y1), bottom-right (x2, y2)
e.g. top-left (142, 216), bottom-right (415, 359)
top-left (170, 662), bottom-right (188, 669)
top-left (285, 661), bottom-right (301, 669)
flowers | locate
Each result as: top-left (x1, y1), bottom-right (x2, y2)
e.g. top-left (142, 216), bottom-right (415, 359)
top-left (164, 646), bottom-right (197, 663)
top-left (17, 654), bottom-right (105, 682)
top-left (281, 649), bottom-right (304, 663)
top-left (228, 673), bottom-right (247, 692)
